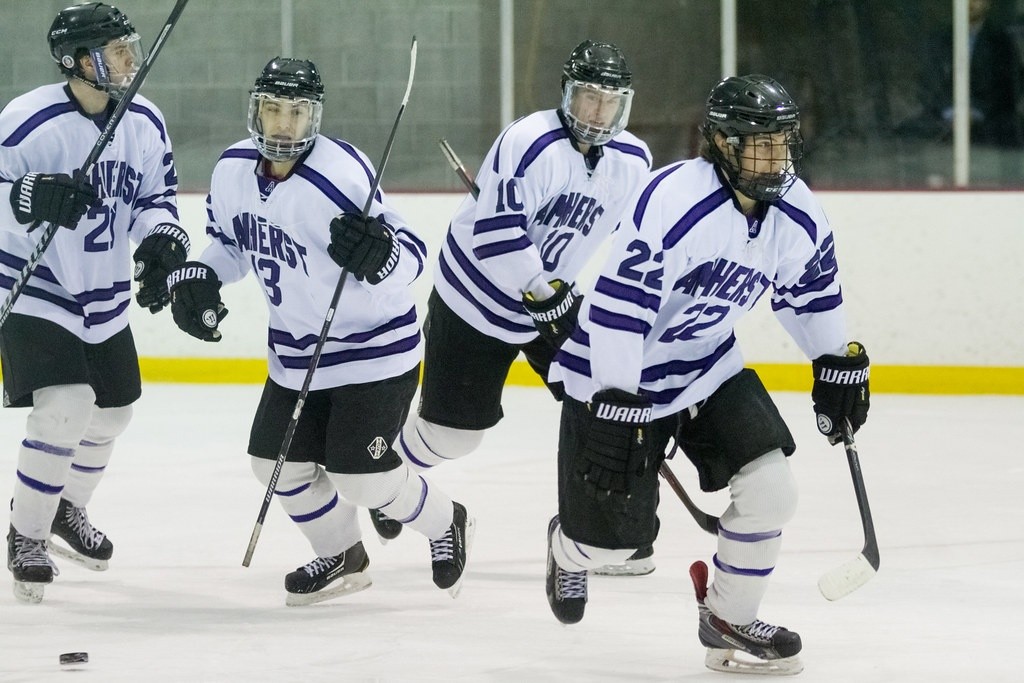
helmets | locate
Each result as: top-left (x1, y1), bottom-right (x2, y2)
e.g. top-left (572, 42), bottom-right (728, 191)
top-left (559, 38), bottom-right (635, 146)
top-left (246, 56), bottom-right (324, 162)
top-left (47, 1), bottom-right (147, 99)
top-left (697, 74), bottom-right (803, 201)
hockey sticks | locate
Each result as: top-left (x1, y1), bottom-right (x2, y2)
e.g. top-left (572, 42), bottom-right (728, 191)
top-left (817, 408), bottom-right (883, 605)
top-left (435, 135), bottom-right (727, 536)
top-left (234, 30), bottom-right (422, 574)
top-left (0, 0), bottom-right (191, 334)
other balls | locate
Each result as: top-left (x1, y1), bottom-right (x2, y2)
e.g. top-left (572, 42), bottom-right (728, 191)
top-left (59, 651), bottom-right (89, 664)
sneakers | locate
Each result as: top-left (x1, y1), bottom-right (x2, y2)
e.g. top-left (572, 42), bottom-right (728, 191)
top-left (547, 513), bottom-right (589, 624)
top-left (285, 541), bottom-right (372, 606)
top-left (5, 521), bottom-right (59, 605)
top-left (586, 516), bottom-right (660, 576)
top-left (48, 499), bottom-right (114, 571)
top-left (367, 507), bottom-right (402, 543)
top-left (690, 561), bottom-right (804, 676)
top-left (428, 499), bottom-right (472, 598)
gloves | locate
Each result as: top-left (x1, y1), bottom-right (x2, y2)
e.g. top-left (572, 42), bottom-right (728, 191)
top-left (10, 172), bottom-right (98, 230)
top-left (521, 278), bottom-right (583, 350)
top-left (578, 388), bottom-right (652, 500)
top-left (165, 261), bottom-right (228, 343)
top-left (133, 222), bottom-right (191, 314)
top-left (811, 341), bottom-right (871, 446)
top-left (327, 211), bottom-right (400, 285)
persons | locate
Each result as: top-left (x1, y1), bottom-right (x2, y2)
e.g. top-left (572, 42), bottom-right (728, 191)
top-left (166, 55), bottom-right (475, 608)
top-left (889, 0), bottom-right (1024, 153)
top-left (364, 44), bottom-right (663, 577)
top-left (0, 0), bottom-right (191, 601)
top-left (544, 70), bottom-right (871, 677)
